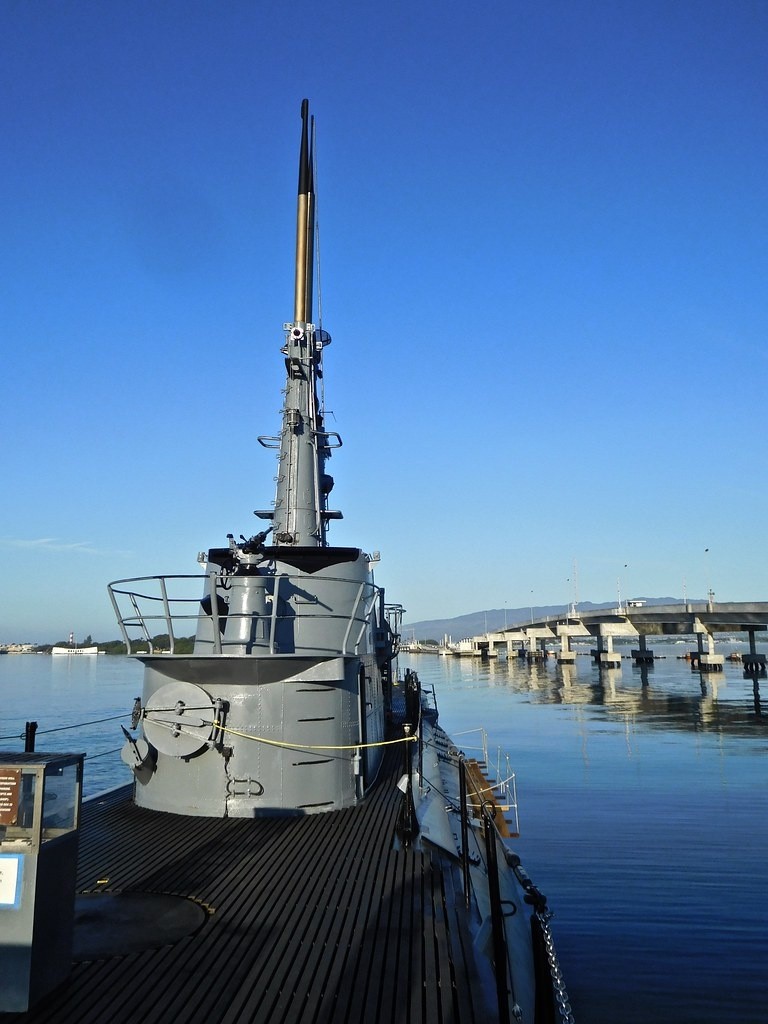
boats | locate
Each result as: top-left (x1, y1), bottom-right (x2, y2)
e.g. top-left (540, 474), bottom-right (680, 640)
top-left (725, 651), bottom-right (744, 663)
top-left (676, 650), bottom-right (691, 659)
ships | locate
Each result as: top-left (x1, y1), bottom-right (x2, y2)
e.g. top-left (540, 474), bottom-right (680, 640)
top-left (0, 86), bottom-right (585, 1024)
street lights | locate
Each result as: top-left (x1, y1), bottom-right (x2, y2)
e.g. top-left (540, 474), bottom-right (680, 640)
top-left (482, 544), bottom-right (717, 632)
